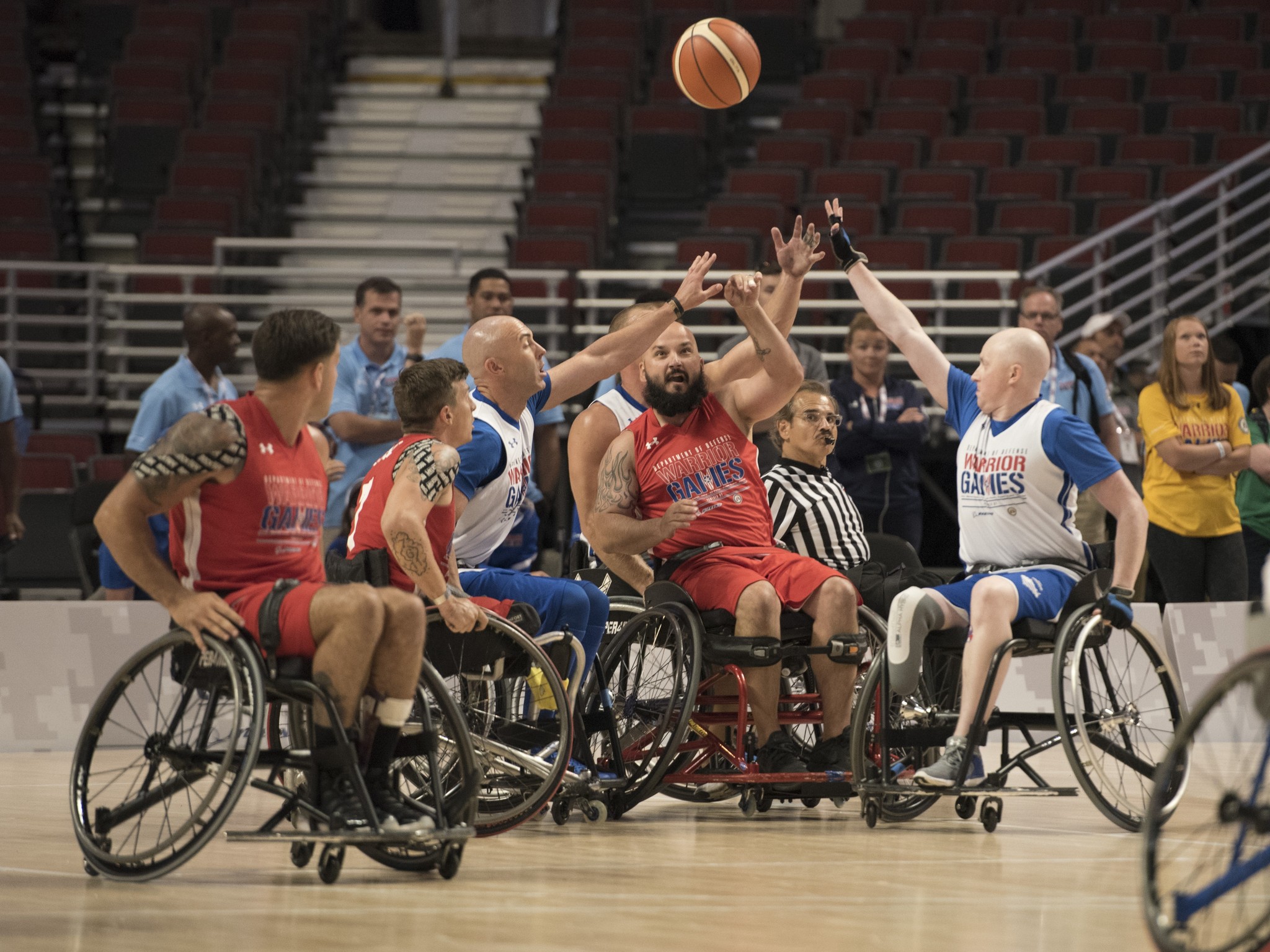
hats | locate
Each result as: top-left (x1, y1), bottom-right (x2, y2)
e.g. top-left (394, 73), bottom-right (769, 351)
top-left (1082, 311), bottom-right (1133, 338)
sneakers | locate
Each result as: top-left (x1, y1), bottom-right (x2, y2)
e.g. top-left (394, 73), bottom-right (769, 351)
top-left (364, 767), bottom-right (436, 833)
top-left (913, 735), bottom-right (990, 788)
top-left (755, 729), bottom-right (812, 792)
top-left (808, 723), bottom-right (880, 779)
top-left (529, 745), bottom-right (616, 781)
top-left (320, 777), bottom-right (398, 830)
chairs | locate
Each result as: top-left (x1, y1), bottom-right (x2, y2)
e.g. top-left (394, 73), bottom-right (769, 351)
top-left (0, 0), bottom-right (1270, 497)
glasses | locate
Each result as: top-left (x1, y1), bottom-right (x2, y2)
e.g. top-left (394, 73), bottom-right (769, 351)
top-left (1021, 308), bottom-right (1059, 323)
top-left (791, 412), bottom-right (844, 427)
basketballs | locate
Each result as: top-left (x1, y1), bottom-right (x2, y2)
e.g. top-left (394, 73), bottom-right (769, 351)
top-left (672, 17), bottom-right (761, 109)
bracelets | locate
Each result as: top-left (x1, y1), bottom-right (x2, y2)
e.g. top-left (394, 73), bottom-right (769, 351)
top-left (404, 352), bottom-right (424, 364)
top-left (665, 297), bottom-right (684, 319)
top-left (434, 588), bottom-right (451, 606)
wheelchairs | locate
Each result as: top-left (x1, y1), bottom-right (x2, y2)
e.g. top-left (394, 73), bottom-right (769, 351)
top-left (265, 522), bottom-right (962, 840)
top-left (1140, 639), bottom-right (1269, 952)
top-left (67, 616), bottom-right (484, 887)
top-left (850, 534), bottom-right (1199, 833)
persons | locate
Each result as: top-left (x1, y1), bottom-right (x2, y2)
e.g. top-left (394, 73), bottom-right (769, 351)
top-left (434, 265), bottom-right (561, 570)
top-left (434, 249), bottom-right (722, 787)
top-left (1006, 287), bottom-right (1270, 603)
top-left (821, 190), bottom-right (1150, 785)
top-left (0, 349), bottom-right (29, 601)
top-left (71, 303), bottom-right (242, 609)
top-left (92, 307), bottom-right (429, 856)
top-left (571, 199), bottom-right (962, 775)
top-left (316, 275), bottom-right (428, 560)
top-left (343, 356), bottom-right (596, 780)
